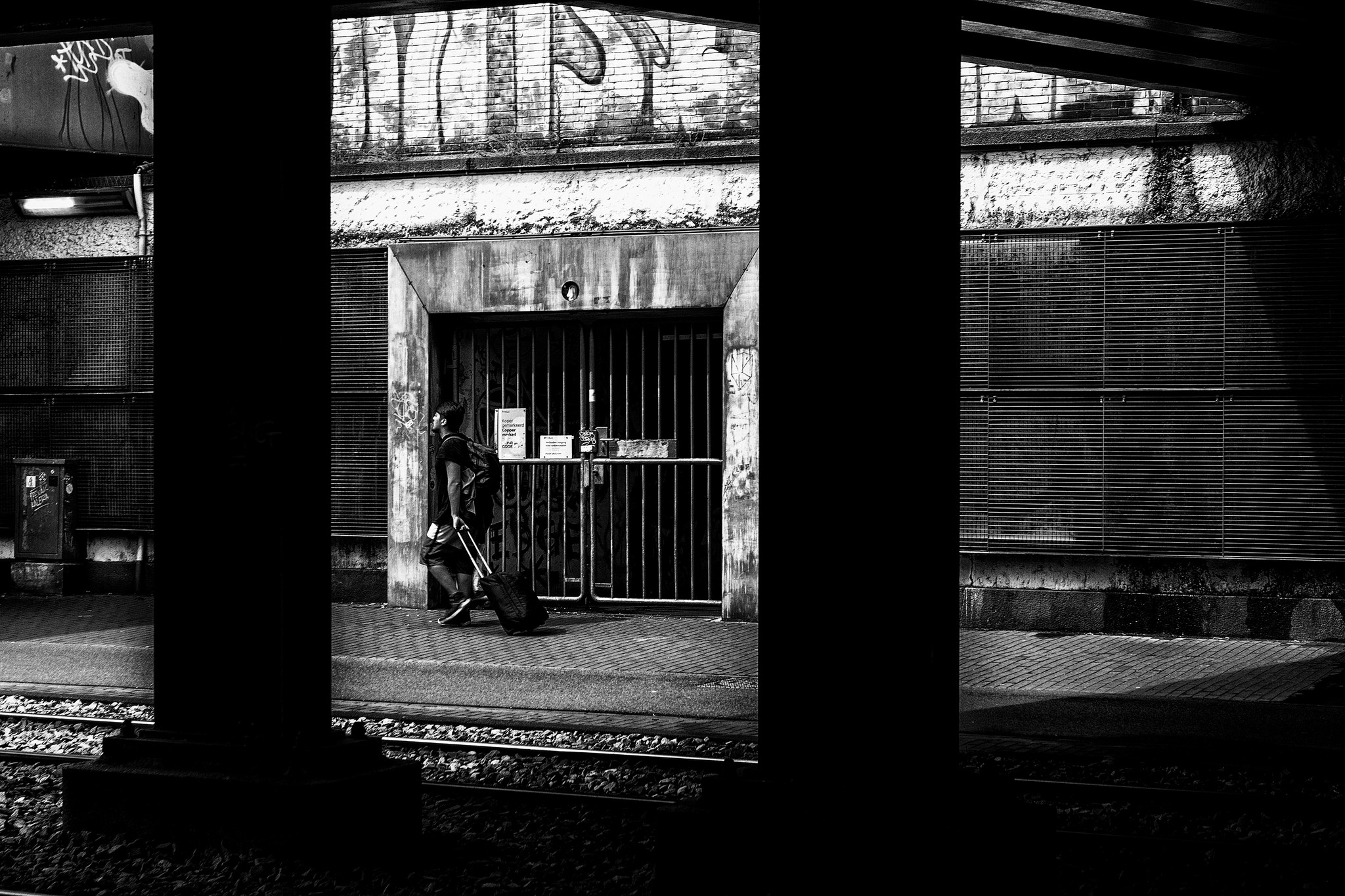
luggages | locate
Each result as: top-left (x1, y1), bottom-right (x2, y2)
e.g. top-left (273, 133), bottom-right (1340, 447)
top-left (455, 528), bottom-right (550, 634)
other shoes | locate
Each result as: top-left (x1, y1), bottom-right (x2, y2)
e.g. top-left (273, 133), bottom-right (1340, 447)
top-left (437, 616), bottom-right (472, 626)
top-left (443, 595), bottom-right (471, 621)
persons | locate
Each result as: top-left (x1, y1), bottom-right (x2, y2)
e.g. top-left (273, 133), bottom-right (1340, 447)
top-left (418, 403), bottom-right (501, 626)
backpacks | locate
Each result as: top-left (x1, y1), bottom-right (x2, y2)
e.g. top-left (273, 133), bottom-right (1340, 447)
top-left (439, 436), bottom-right (501, 495)
